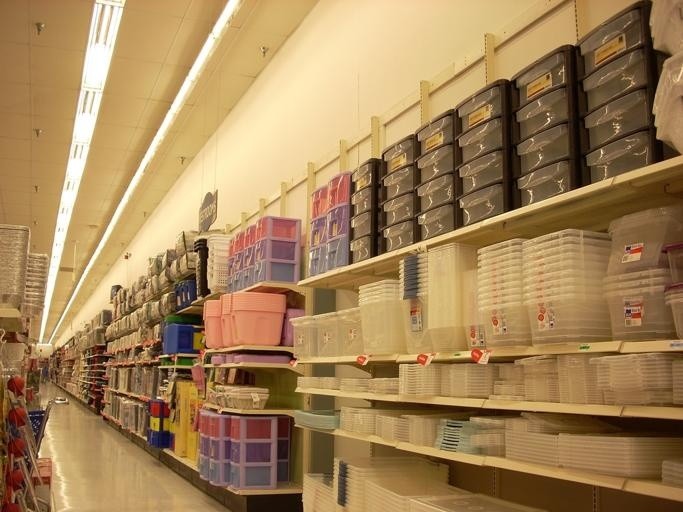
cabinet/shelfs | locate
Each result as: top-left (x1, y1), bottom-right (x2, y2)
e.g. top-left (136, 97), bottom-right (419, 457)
top-left (52, 344), bottom-right (107, 415)
top-left (157, 280), bottom-right (311, 512)
top-left (295, 151), bottom-right (683, 512)
top-left (100, 286), bottom-right (174, 460)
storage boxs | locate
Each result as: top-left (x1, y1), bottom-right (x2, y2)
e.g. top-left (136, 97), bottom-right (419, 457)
top-left (409, 493), bottom-right (547, 512)
top-left (348, 157), bottom-right (379, 266)
top-left (312, 311), bottom-right (338, 358)
top-left (661, 241), bottom-right (683, 340)
top-left (357, 279), bottom-right (407, 355)
top-left (453, 78), bottom-right (512, 230)
top-left (522, 228), bottom-right (611, 344)
top-left (364, 478), bottom-right (472, 512)
top-left (307, 184), bottom-right (326, 277)
top-left (225, 216), bottom-right (302, 293)
top-left (197, 411), bottom-right (215, 481)
top-left (414, 109), bottom-right (453, 244)
top-left (288, 315), bottom-right (317, 359)
top-left (301, 472), bottom-right (333, 512)
top-left (398, 252), bottom-right (434, 354)
top-left (507, 43), bottom-right (581, 210)
top-left (603, 204), bottom-right (683, 340)
top-left (573, 1), bottom-right (663, 186)
top-left (337, 307), bottom-right (364, 356)
top-left (333, 456), bottom-right (449, 512)
top-left (219, 293), bottom-right (231, 347)
top-left (325, 171), bottom-right (351, 270)
top-left (427, 242), bottom-right (468, 351)
top-left (476, 238), bottom-right (531, 345)
top-left (207, 414), bottom-right (230, 487)
top-left (229, 293), bottom-right (287, 346)
top-left (229, 415), bottom-right (278, 490)
top-left (277, 416), bottom-right (291, 482)
top-left (202, 299), bottom-right (223, 349)
top-left (379, 134), bottom-right (414, 256)
